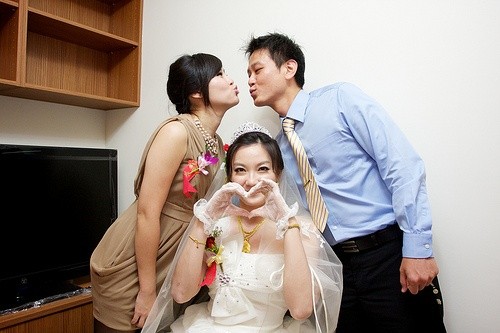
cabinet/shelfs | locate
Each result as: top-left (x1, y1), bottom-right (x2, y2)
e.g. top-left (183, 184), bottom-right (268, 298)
top-left (0, 0), bottom-right (144, 110)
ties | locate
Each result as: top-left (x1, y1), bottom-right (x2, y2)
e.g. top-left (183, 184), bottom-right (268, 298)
top-left (283, 118), bottom-right (328, 233)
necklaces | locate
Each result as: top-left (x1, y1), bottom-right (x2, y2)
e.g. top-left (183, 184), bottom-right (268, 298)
top-left (190, 114), bottom-right (219, 155)
top-left (237, 216), bottom-right (266, 253)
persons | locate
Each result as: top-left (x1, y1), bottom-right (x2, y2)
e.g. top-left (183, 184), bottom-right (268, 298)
top-left (90, 53), bottom-right (240, 333)
top-left (244, 31), bottom-right (446, 333)
top-left (141, 120), bottom-right (344, 333)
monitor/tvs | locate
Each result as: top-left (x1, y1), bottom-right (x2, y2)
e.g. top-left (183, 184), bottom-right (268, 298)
top-left (0, 144), bottom-right (118, 314)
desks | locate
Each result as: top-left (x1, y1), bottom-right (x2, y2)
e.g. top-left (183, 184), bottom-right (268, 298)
top-left (0, 274), bottom-right (94, 333)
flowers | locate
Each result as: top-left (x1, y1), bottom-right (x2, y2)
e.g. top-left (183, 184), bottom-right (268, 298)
top-left (204, 226), bottom-right (224, 274)
top-left (187, 143), bottom-right (219, 175)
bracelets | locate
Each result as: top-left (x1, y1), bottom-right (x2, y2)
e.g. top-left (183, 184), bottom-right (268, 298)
top-left (188, 235), bottom-right (206, 248)
top-left (283, 224), bottom-right (300, 230)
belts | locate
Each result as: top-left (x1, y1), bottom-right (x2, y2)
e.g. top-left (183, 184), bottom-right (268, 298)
top-left (333, 223), bottom-right (402, 256)
